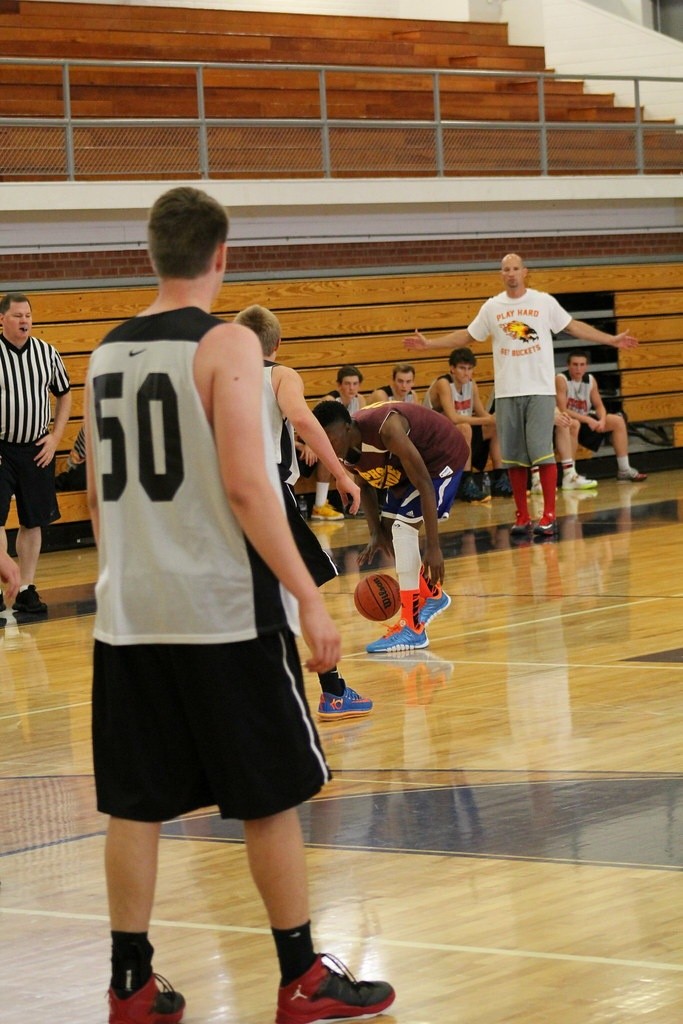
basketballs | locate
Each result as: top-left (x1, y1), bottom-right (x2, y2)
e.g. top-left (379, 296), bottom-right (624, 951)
top-left (353, 573), bottom-right (402, 621)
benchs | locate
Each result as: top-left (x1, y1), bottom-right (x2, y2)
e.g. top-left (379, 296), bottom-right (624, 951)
top-left (0, 0), bottom-right (683, 182)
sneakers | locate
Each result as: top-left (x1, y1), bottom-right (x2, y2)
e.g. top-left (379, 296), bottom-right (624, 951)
top-left (618, 467), bottom-right (647, 481)
top-left (456, 470), bottom-right (491, 503)
top-left (0, 592), bottom-right (6, 613)
top-left (530, 477), bottom-right (541, 494)
top-left (561, 470), bottom-right (597, 490)
top-left (310, 502), bottom-right (344, 520)
top-left (108, 969), bottom-right (185, 1024)
top-left (276, 953), bottom-right (395, 1024)
top-left (12, 583), bottom-right (47, 611)
top-left (534, 515), bottom-right (558, 535)
top-left (366, 621), bottom-right (429, 653)
top-left (317, 686), bottom-right (374, 720)
top-left (418, 577), bottom-right (451, 627)
top-left (488, 470), bottom-right (514, 498)
top-left (510, 510), bottom-right (532, 535)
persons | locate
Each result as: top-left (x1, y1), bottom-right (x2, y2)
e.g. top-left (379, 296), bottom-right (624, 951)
top-left (432, 348), bottom-right (647, 505)
top-left (232, 305), bottom-right (374, 722)
top-left (286, 365), bottom-right (420, 521)
top-left (403, 253), bottom-right (640, 535)
top-left (313, 399), bottom-right (469, 653)
top-left (0, 293), bottom-right (73, 627)
top-left (85, 187), bottom-right (397, 1024)
